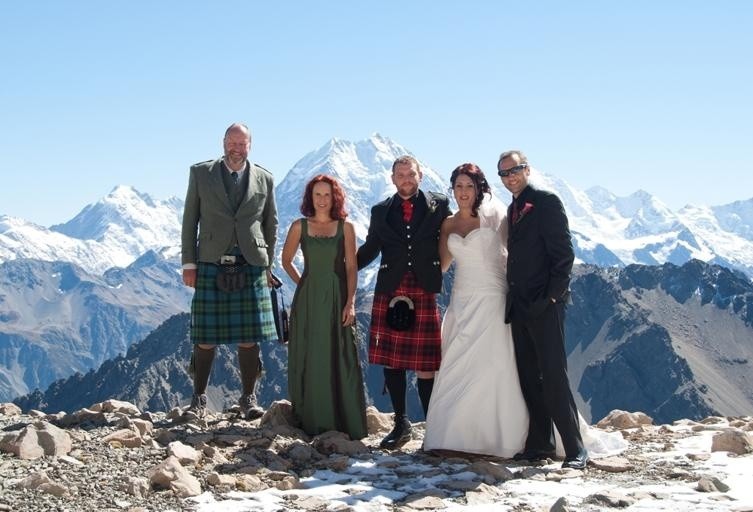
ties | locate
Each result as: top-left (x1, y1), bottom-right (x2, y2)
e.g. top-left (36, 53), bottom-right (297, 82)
top-left (231, 172), bottom-right (239, 210)
top-left (512, 201), bottom-right (519, 225)
top-left (402, 200), bottom-right (414, 222)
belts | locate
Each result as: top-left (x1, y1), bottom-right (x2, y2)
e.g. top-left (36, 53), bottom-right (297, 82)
top-left (218, 254), bottom-right (246, 266)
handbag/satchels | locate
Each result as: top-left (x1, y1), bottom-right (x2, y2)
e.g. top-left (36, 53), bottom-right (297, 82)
top-left (271, 285), bottom-right (288, 344)
top-left (216, 265), bottom-right (245, 292)
top-left (386, 296), bottom-right (416, 331)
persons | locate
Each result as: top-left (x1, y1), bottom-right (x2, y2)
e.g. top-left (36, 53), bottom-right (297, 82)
top-left (420, 163), bottom-right (589, 458)
top-left (281, 172), bottom-right (368, 442)
top-left (357, 156), bottom-right (453, 449)
top-left (497, 149), bottom-right (590, 469)
top-left (180, 122), bottom-right (279, 416)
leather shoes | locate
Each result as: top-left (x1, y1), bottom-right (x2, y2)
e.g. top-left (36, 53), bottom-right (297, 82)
top-left (562, 448), bottom-right (590, 468)
top-left (380, 420), bottom-right (411, 449)
top-left (514, 448), bottom-right (557, 462)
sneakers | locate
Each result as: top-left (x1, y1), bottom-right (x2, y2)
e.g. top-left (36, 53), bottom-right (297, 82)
top-left (238, 394), bottom-right (264, 419)
top-left (182, 395), bottom-right (207, 419)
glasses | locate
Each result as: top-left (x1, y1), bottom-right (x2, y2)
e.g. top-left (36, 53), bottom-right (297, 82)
top-left (498, 164), bottom-right (527, 176)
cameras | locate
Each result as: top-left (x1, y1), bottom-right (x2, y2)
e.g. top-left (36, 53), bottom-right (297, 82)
top-left (272, 273), bottom-right (282, 289)
